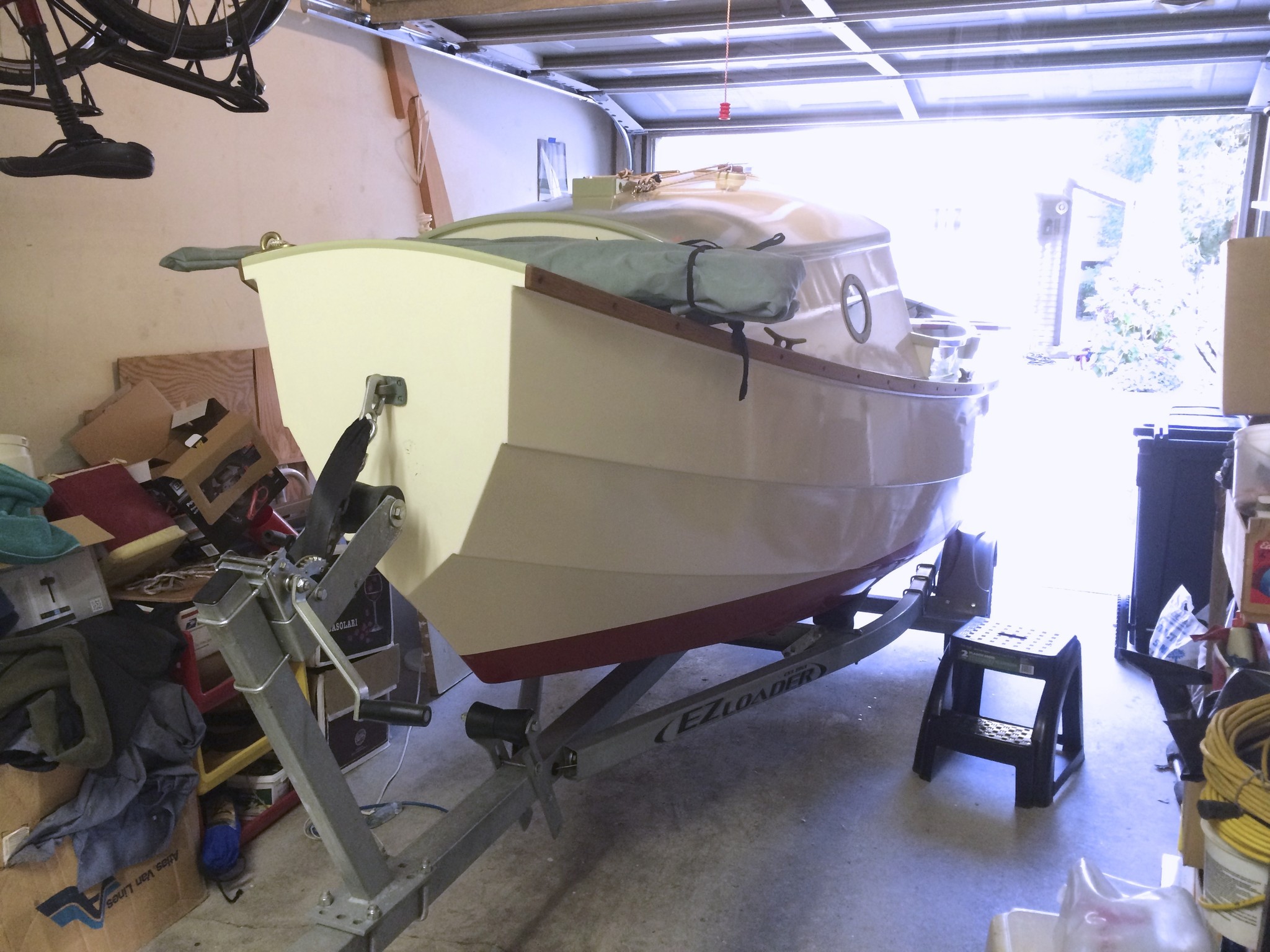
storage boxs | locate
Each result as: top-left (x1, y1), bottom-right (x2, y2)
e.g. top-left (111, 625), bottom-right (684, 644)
top-left (1, 514), bottom-right (111, 641)
top-left (303, 644), bottom-right (391, 775)
top-left (1221, 489), bottom-right (1270, 625)
top-left (70, 379), bottom-right (288, 564)
top-left (305, 546), bottom-right (394, 669)
top-left (0, 738), bottom-right (209, 951)
top-left (136, 603), bottom-right (220, 666)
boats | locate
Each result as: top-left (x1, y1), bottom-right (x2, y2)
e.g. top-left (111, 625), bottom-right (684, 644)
top-left (152, 159), bottom-right (1008, 952)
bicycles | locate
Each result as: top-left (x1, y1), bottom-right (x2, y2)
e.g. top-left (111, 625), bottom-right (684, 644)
top-left (0, 0), bottom-right (291, 181)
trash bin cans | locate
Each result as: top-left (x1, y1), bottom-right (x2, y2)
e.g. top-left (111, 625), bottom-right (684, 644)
top-left (1108, 410), bottom-right (1253, 659)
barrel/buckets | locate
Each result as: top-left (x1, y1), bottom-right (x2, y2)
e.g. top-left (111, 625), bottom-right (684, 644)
top-left (1233, 416), bottom-right (1270, 518)
top-left (1197, 812), bottom-right (1269, 952)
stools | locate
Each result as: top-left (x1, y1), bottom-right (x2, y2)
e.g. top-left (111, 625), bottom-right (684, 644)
top-left (914, 615), bottom-right (1086, 811)
